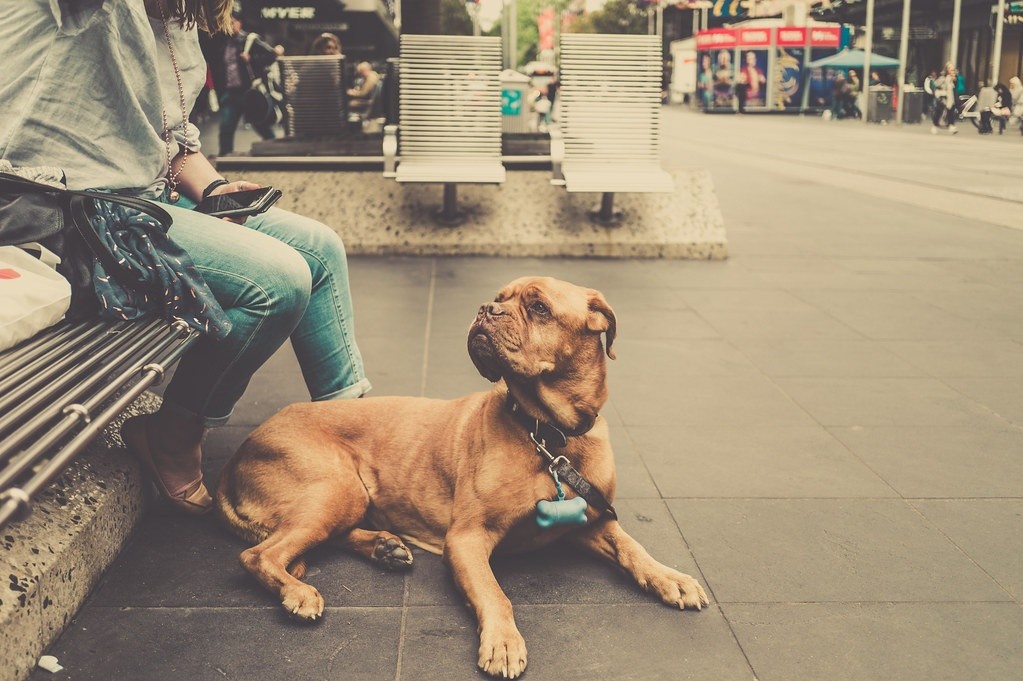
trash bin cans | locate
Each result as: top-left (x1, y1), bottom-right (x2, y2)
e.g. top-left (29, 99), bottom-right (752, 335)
top-left (866, 90), bottom-right (894, 123)
top-left (901, 91), bottom-right (925, 124)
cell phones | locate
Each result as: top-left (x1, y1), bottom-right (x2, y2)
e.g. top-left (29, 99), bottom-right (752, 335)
top-left (192, 187), bottom-right (275, 218)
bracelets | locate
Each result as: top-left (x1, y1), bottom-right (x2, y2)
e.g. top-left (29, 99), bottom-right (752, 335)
top-left (202, 179), bottom-right (229, 198)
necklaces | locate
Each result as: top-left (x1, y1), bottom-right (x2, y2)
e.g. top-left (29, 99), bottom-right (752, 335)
top-left (159, 2), bottom-right (188, 201)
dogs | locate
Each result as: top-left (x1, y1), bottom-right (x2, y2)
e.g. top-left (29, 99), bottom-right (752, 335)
top-left (212, 275), bottom-right (710, 681)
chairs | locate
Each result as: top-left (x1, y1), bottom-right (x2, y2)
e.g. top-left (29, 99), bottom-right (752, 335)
top-left (557, 32), bottom-right (676, 227)
top-left (383, 32), bottom-right (504, 228)
top-left (276, 52), bottom-right (363, 139)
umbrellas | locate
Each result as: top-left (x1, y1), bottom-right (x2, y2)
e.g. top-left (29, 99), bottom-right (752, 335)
top-left (804, 45), bottom-right (899, 68)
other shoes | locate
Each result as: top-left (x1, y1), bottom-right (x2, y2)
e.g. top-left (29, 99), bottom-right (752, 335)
top-left (120, 415), bottom-right (213, 516)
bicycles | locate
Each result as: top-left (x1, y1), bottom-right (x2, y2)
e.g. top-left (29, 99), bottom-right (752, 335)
top-left (954, 94), bottom-right (994, 128)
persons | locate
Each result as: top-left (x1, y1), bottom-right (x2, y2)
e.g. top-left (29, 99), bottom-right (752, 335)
top-left (535, 82), bottom-right (562, 132)
top-left (979, 76), bottom-right (1023, 134)
top-left (832, 69), bottom-right (890, 124)
top-left (0, 1), bottom-right (372, 514)
top-left (924, 60), bottom-right (965, 135)
top-left (206, 1), bottom-right (279, 158)
top-left (285, 33), bottom-right (379, 99)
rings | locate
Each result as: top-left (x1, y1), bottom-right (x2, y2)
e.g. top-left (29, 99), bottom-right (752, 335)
top-left (240, 182), bottom-right (246, 190)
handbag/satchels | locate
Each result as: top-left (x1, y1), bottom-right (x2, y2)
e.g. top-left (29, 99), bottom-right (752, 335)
top-left (0, 242), bottom-right (71, 352)
top-left (246, 77), bottom-right (271, 111)
top-left (0, 172), bottom-right (173, 321)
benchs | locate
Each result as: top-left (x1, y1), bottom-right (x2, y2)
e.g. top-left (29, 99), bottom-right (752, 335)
top-left (0, 312), bottom-right (199, 681)
top-left (962, 86), bottom-right (1023, 135)
